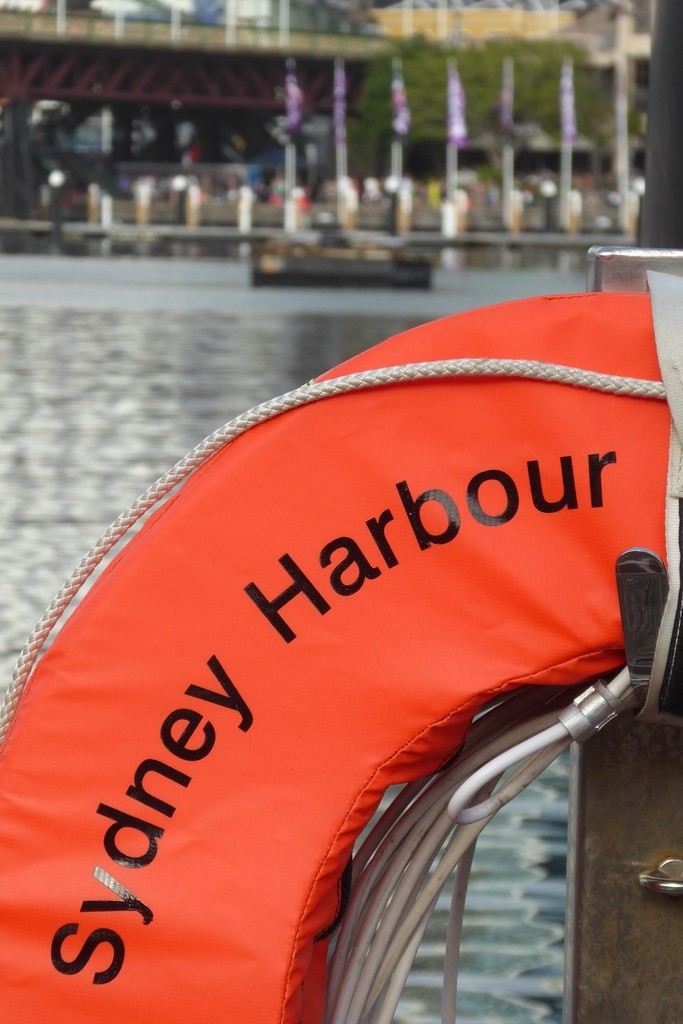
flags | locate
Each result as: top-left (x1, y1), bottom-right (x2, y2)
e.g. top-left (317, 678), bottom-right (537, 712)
top-left (499, 55), bottom-right (518, 143)
top-left (445, 57), bottom-right (468, 148)
top-left (333, 52), bottom-right (348, 143)
top-left (559, 58), bottom-right (579, 141)
top-left (284, 57), bottom-right (303, 143)
top-left (390, 60), bottom-right (410, 140)
